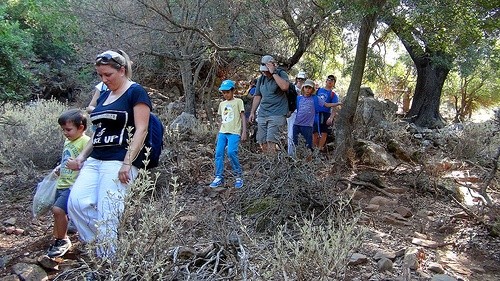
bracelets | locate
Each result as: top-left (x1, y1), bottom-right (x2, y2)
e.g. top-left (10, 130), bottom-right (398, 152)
top-left (123, 162), bottom-right (130, 165)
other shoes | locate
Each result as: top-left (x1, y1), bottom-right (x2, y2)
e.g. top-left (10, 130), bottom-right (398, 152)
top-left (234, 178), bottom-right (244, 188)
top-left (209, 175), bottom-right (225, 188)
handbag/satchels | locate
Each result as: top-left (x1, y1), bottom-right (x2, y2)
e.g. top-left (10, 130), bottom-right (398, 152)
top-left (32, 165), bottom-right (60, 218)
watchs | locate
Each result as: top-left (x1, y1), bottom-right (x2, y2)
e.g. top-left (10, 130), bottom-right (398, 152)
top-left (272, 70), bottom-right (278, 75)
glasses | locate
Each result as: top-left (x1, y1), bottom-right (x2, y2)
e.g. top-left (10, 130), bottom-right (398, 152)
top-left (327, 78), bottom-right (335, 82)
top-left (261, 62), bottom-right (266, 66)
top-left (96, 54), bottom-right (123, 66)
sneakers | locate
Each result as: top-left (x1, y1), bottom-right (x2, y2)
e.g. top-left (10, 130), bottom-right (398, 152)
top-left (48, 237), bottom-right (72, 258)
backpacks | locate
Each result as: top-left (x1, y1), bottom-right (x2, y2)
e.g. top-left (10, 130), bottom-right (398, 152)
top-left (98, 83), bottom-right (164, 170)
top-left (277, 69), bottom-right (298, 111)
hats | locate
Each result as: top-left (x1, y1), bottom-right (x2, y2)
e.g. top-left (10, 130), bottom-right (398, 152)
top-left (303, 79), bottom-right (315, 89)
top-left (328, 75), bottom-right (336, 82)
top-left (297, 72), bottom-right (308, 79)
top-left (260, 55), bottom-right (274, 71)
top-left (219, 80), bottom-right (235, 91)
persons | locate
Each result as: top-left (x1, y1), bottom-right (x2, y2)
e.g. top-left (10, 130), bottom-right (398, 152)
top-left (208, 80), bottom-right (247, 188)
top-left (286, 72), bottom-right (307, 151)
top-left (66, 49), bottom-right (152, 258)
top-left (313, 75), bottom-right (339, 149)
top-left (46, 110), bottom-right (92, 257)
top-left (243, 77), bottom-right (257, 95)
top-left (87, 80), bottom-right (108, 112)
top-left (249, 55), bottom-right (290, 154)
top-left (288, 79), bottom-right (343, 151)
